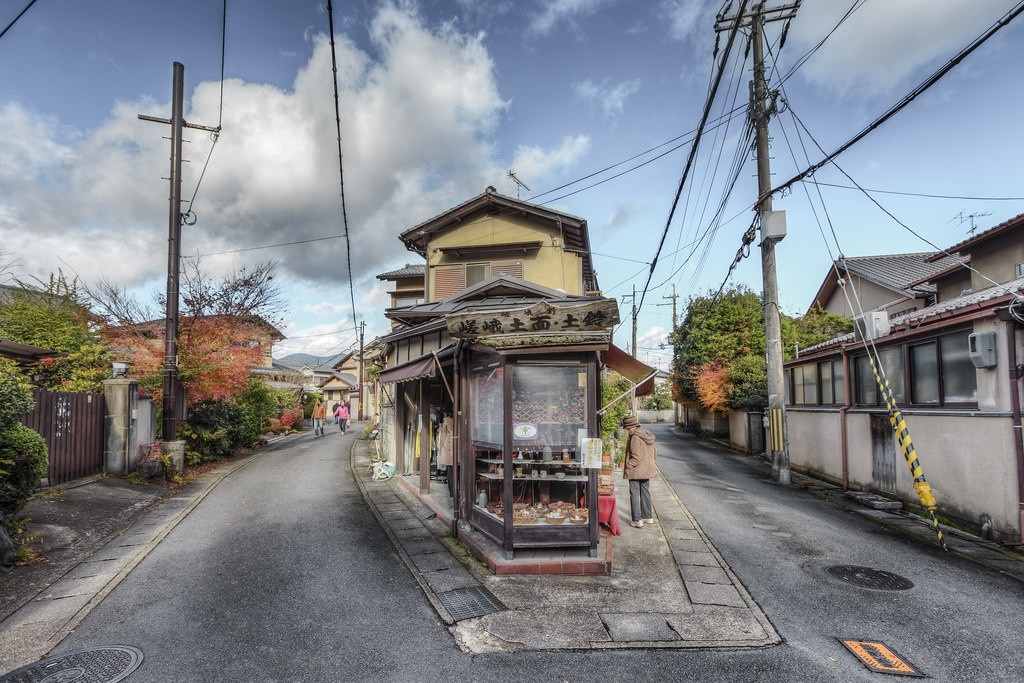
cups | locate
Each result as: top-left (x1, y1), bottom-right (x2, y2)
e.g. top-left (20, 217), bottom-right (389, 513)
top-left (532, 470), bottom-right (538, 478)
top-left (516, 468), bottom-right (523, 478)
top-left (540, 470), bottom-right (547, 479)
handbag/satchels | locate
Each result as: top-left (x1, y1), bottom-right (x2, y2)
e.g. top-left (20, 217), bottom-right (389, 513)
top-left (347, 420), bottom-right (350, 429)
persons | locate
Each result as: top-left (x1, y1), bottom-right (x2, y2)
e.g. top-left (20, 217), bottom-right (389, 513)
top-left (439, 409), bottom-right (460, 497)
top-left (332, 400), bottom-right (350, 435)
top-left (623, 415), bottom-right (657, 527)
top-left (311, 398), bottom-right (327, 439)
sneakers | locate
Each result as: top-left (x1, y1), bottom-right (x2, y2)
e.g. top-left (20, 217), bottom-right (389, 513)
top-left (642, 518), bottom-right (654, 524)
top-left (629, 520), bottom-right (644, 527)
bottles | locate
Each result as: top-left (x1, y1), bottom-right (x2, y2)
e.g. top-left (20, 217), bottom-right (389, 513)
top-left (479, 490), bottom-right (487, 508)
top-left (563, 449), bottom-right (569, 463)
top-left (543, 445), bottom-right (552, 462)
top-left (518, 451), bottom-right (523, 462)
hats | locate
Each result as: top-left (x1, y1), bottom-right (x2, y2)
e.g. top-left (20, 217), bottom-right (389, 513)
top-left (623, 416), bottom-right (639, 428)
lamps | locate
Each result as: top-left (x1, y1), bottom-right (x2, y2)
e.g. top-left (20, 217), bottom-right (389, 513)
top-left (110, 361), bottom-right (133, 378)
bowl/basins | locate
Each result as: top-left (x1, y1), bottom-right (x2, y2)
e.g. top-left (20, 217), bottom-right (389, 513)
top-left (485, 501), bottom-right (588, 524)
top-left (555, 473), bottom-right (565, 479)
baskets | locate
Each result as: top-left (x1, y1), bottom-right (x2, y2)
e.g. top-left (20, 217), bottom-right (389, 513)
top-left (487, 500), bottom-right (588, 525)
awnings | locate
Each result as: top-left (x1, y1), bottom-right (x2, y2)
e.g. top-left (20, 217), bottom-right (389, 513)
top-left (598, 343), bottom-right (659, 415)
top-left (377, 340), bottom-right (462, 416)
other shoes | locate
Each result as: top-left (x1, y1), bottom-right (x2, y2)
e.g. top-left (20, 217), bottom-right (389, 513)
top-left (321, 434), bottom-right (324, 437)
top-left (315, 435), bottom-right (319, 439)
top-left (342, 432), bottom-right (345, 436)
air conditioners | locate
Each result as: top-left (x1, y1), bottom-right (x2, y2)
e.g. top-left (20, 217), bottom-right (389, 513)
top-left (854, 311), bottom-right (888, 344)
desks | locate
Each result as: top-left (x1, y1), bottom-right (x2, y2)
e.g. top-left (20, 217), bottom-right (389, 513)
top-left (581, 488), bottom-right (620, 535)
